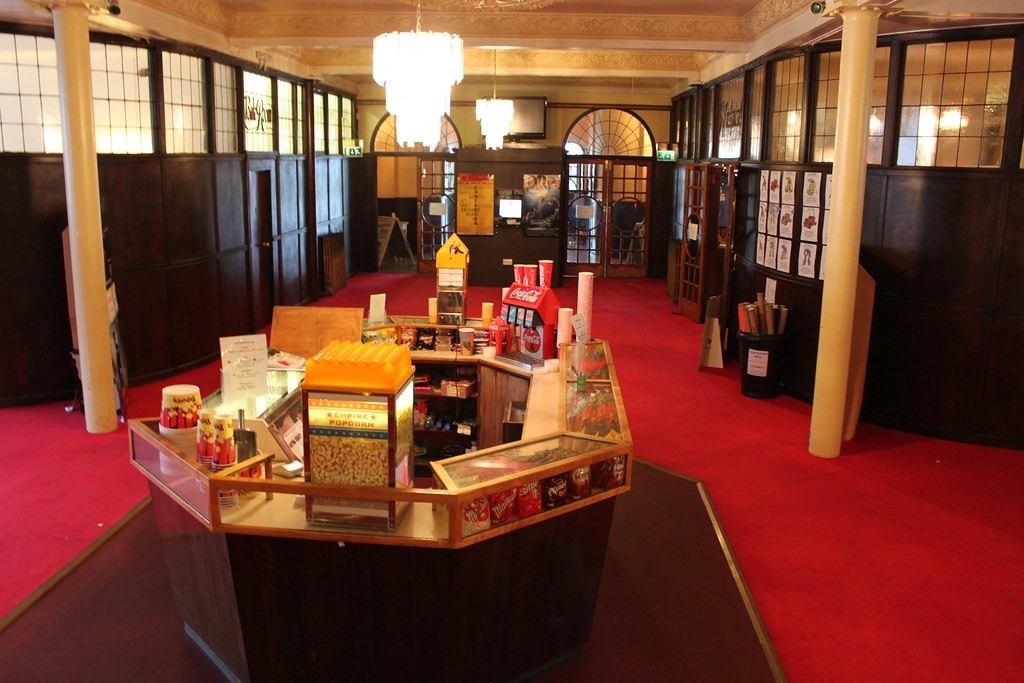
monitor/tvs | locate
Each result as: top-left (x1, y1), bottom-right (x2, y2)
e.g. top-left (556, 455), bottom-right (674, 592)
top-left (499, 199), bottom-right (522, 219)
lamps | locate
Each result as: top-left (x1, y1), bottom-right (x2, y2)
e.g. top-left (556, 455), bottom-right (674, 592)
top-left (373, 0), bottom-right (464, 153)
top-left (476, 49), bottom-right (514, 151)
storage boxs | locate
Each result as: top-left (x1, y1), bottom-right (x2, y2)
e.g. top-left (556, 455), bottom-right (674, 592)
top-left (301, 369), bottom-right (416, 530)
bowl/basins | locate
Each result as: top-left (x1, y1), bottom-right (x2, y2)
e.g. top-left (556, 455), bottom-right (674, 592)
top-left (237, 449), bottom-right (263, 500)
top-left (159, 384), bottom-right (204, 476)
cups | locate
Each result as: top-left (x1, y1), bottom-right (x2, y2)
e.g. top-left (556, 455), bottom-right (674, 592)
top-left (524, 265), bottom-right (538, 285)
top-left (514, 264), bottom-right (524, 284)
top-left (197, 410), bottom-right (237, 509)
top-left (539, 260), bottom-right (553, 288)
top-left (459, 328), bottom-right (475, 356)
top-left (557, 308), bottom-right (573, 360)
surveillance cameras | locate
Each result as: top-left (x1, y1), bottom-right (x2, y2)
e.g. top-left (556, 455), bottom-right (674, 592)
top-left (811, 2), bottom-right (825, 14)
top-left (107, 0), bottom-right (121, 14)
